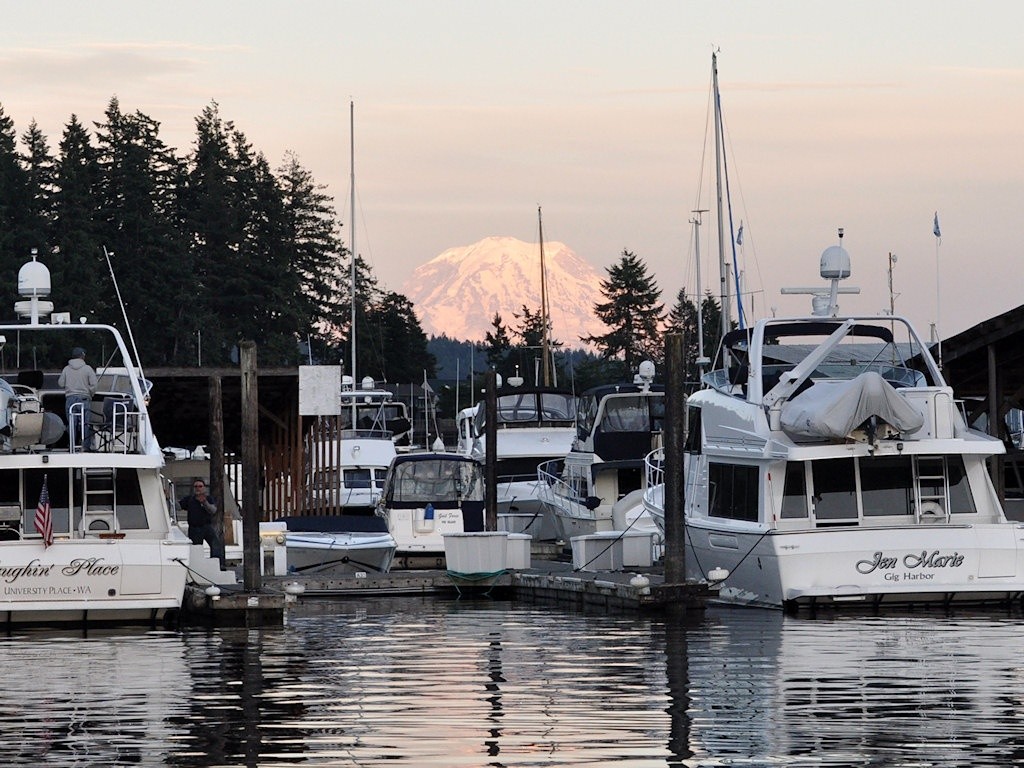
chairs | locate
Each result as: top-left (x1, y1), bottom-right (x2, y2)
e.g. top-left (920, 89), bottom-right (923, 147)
top-left (83, 397), bottom-right (137, 455)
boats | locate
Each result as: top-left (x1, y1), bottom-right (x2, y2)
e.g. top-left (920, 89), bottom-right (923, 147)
top-left (1, 243), bottom-right (239, 636)
top-left (639, 226), bottom-right (1023, 619)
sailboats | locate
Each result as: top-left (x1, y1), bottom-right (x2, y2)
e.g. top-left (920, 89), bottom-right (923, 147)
top-left (170, 56), bottom-right (1022, 557)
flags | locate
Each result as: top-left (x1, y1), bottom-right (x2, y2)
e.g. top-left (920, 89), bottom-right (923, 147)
top-left (33, 478), bottom-right (53, 550)
top-left (737, 226), bottom-right (743, 245)
top-left (933, 215), bottom-right (941, 237)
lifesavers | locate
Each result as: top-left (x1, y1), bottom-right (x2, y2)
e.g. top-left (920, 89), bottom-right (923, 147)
top-left (913, 501), bottom-right (946, 524)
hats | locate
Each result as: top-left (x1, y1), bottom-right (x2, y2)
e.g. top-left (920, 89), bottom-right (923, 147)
top-left (71, 347), bottom-right (88, 360)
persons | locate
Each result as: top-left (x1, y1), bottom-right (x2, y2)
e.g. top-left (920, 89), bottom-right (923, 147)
top-left (163, 480), bottom-right (226, 571)
top-left (58, 347), bottom-right (98, 453)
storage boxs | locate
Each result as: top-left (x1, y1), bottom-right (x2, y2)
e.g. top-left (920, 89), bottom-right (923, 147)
top-left (570, 534), bottom-right (624, 572)
top-left (506, 533), bottom-right (533, 570)
top-left (594, 532), bottom-right (656, 566)
top-left (441, 530), bottom-right (508, 572)
top-left (496, 513), bottom-right (544, 541)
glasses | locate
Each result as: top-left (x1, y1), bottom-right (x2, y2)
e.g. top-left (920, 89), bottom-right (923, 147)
top-left (194, 485), bottom-right (203, 488)
